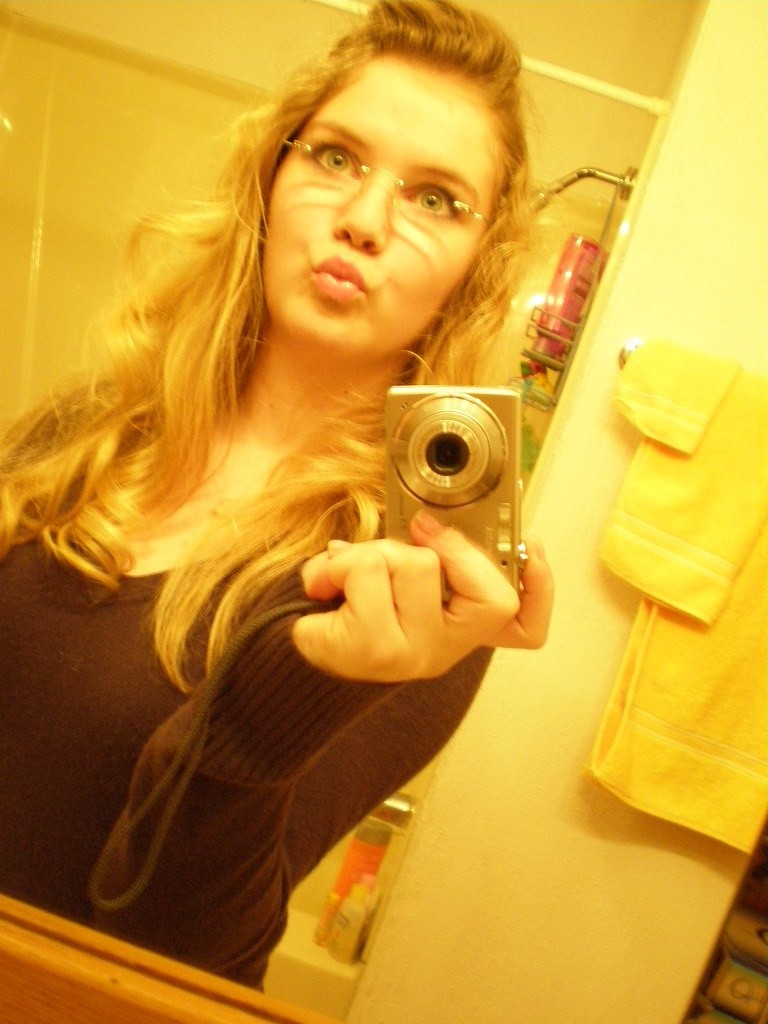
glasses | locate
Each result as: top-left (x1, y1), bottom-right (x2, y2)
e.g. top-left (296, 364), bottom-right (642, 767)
top-left (283, 129), bottom-right (491, 250)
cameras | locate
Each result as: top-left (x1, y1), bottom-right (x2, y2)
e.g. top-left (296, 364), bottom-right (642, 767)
top-left (386, 383), bottom-right (527, 603)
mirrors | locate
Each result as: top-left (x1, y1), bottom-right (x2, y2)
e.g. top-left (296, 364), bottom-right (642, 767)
top-left (0, 0), bottom-right (707, 1023)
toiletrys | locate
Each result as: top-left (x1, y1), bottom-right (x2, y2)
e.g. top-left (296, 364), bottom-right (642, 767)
top-left (530, 232), bottom-right (609, 362)
top-left (314, 817), bottom-right (394, 965)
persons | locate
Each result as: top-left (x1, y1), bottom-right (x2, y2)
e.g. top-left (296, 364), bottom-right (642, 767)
top-left (0, 0), bottom-right (554, 990)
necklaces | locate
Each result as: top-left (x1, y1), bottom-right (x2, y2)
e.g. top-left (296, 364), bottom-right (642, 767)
top-left (205, 478), bottom-right (240, 517)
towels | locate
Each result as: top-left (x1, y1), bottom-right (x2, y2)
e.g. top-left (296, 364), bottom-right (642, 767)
top-left (588, 340), bottom-right (767, 856)
